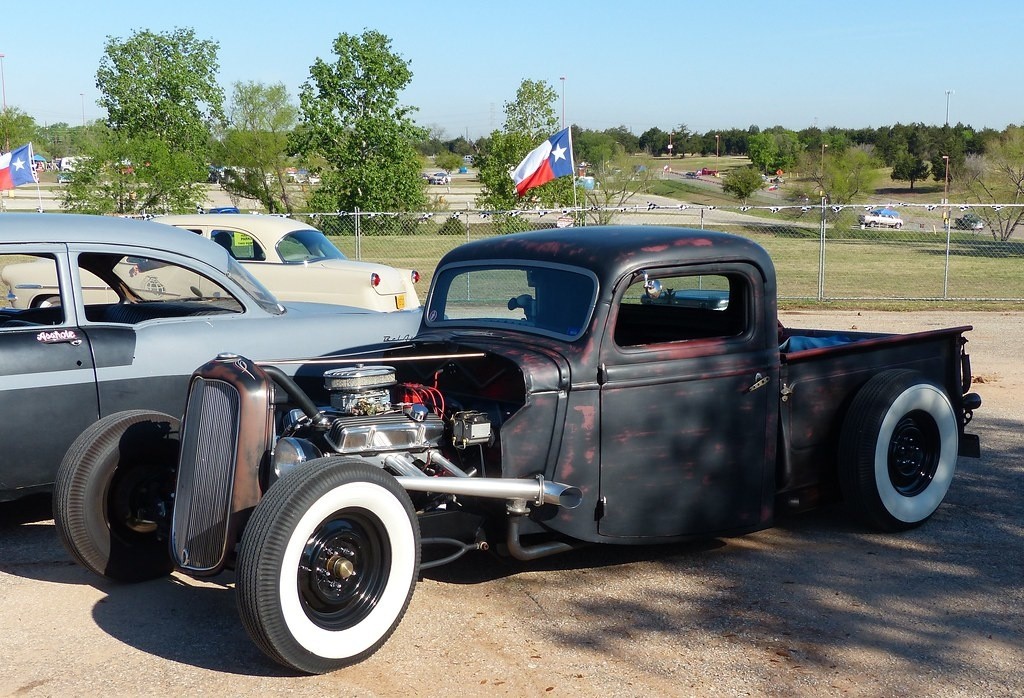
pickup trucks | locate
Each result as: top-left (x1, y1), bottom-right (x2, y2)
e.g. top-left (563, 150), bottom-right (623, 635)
top-left (49, 224), bottom-right (985, 676)
top-left (955, 214), bottom-right (984, 230)
top-left (860, 213), bottom-right (902, 229)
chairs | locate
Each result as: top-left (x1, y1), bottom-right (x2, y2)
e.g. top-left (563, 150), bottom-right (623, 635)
top-left (214, 232), bottom-right (237, 259)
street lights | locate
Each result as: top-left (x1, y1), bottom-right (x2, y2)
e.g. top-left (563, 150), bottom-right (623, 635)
top-left (668, 131), bottom-right (674, 175)
top-left (943, 156), bottom-right (949, 204)
top-left (715, 135), bottom-right (719, 173)
top-left (821, 144), bottom-right (828, 175)
top-left (80, 94), bottom-right (85, 126)
top-left (560, 76), bottom-right (566, 129)
top-left (0, 54), bottom-right (10, 152)
top-left (945, 88), bottom-right (954, 125)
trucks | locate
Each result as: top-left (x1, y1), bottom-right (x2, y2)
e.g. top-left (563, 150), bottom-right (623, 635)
top-left (702, 167), bottom-right (718, 176)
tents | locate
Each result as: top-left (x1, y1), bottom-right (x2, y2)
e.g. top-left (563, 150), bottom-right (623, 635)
top-left (31, 154), bottom-right (47, 172)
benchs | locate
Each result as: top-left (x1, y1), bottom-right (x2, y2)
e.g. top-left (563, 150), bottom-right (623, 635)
top-left (15, 302), bottom-right (239, 324)
top-left (567, 299), bottom-right (745, 346)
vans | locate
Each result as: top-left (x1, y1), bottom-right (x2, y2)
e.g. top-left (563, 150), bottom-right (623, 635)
top-left (60, 157), bottom-right (83, 172)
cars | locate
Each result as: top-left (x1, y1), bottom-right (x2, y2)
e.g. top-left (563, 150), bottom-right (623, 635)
top-left (422, 172), bottom-right (452, 184)
top-left (0, 197), bottom-right (421, 312)
top-left (770, 184), bottom-right (779, 190)
top-left (0, 212), bottom-right (448, 503)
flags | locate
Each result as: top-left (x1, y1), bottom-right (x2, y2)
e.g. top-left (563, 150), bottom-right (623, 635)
top-left (0, 144), bottom-right (36, 190)
top-left (510, 127), bottom-right (573, 198)
